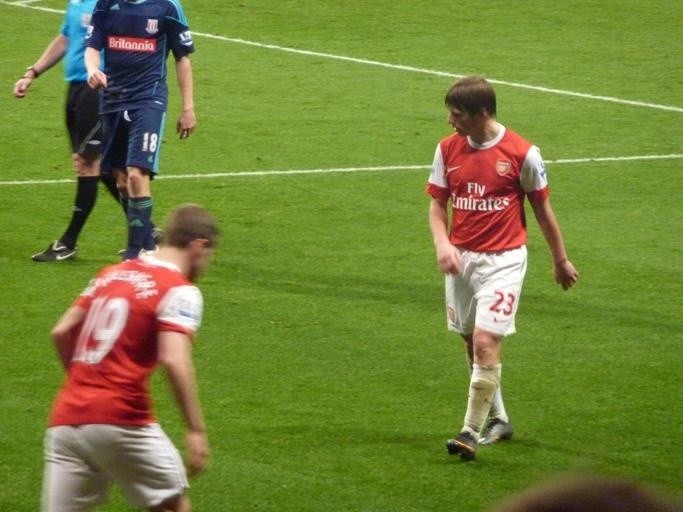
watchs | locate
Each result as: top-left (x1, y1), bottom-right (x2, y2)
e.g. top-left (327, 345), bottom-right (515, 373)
top-left (24, 66), bottom-right (38, 79)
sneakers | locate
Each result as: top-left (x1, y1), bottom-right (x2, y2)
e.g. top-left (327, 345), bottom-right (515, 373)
top-left (477, 417), bottom-right (514, 446)
top-left (120, 224), bottom-right (165, 256)
top-left (30, 238), bottom-right (78, 261)
top-left (446, 430), bottom-right (477, 462)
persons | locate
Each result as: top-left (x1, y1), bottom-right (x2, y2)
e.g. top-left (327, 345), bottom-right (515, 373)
top-left (424, 77), bottom-right (581, 460)
top-left (39, 202), bottom-right (220, 512)
top-left (82, 1), bottom-right (198, 262)
top-left (13, 1), bottom-right (164, 262)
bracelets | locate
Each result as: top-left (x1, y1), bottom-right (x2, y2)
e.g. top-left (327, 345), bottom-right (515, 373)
top-left (20, 75), bottom-right (33, 80)
